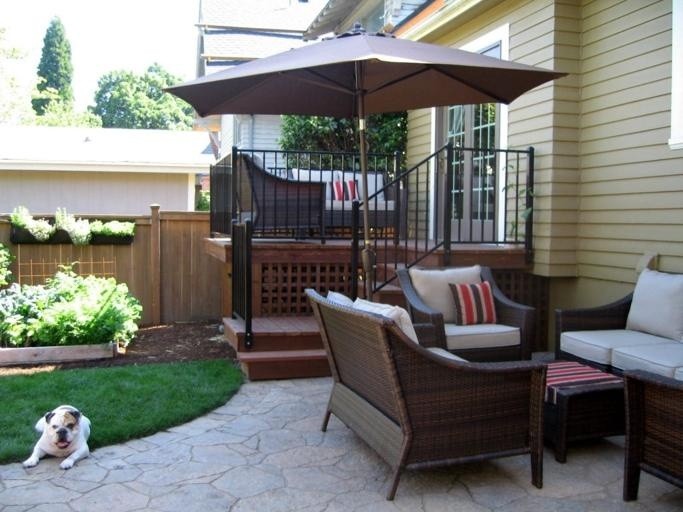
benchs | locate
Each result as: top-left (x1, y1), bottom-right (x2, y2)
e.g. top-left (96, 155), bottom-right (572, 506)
top-left (220, 149), bottom-right (410, 245)
top-left (552, 268), bottom-right (682, 383)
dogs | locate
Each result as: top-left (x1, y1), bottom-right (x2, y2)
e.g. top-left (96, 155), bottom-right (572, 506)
top-left (19, 402), bottom-right (94, 472)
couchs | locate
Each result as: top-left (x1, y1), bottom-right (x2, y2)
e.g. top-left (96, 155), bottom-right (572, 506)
top-left (306, 265), bottom-right (547, 500)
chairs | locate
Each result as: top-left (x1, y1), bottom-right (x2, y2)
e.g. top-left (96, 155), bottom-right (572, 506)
top-left (619, 369), bottom-right (682, 505)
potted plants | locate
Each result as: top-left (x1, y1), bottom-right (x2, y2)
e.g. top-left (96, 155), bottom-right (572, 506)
top-left (0, 205), bottom-right (139, 366)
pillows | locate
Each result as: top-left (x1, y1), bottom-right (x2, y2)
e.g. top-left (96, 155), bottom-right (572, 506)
top-left (624, 268), bottom-right (682, 343)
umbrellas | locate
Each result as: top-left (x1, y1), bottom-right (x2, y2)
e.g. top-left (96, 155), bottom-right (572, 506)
top-left (161, 23), bottom-right (568, 301)
